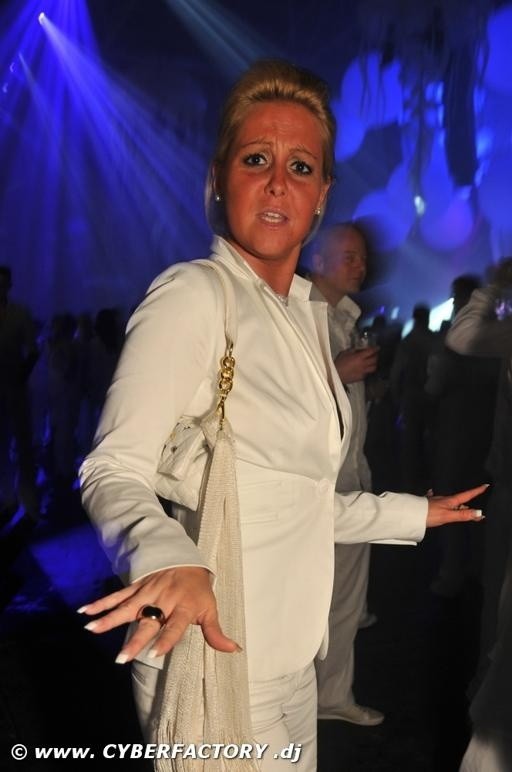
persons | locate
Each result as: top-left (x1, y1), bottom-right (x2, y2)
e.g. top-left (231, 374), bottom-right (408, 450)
top-left (77, 71), bottom-right (491, 772)
top-left (298, 221), bottom-right (385, 727)
top-left (368, 253), bottom-right (512, 772)
top-left (0, 265), bottom-right (139, 611)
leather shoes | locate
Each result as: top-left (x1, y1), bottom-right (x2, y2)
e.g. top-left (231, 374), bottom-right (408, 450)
top-left (317, 703), bottom-right (384, 726)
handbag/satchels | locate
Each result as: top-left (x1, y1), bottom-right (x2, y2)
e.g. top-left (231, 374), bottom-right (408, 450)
top-left (153, 259), bottom-right (235, 512)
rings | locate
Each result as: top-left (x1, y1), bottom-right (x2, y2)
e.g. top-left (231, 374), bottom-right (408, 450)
top-left (136, 603), bottom-right (167, 630)
